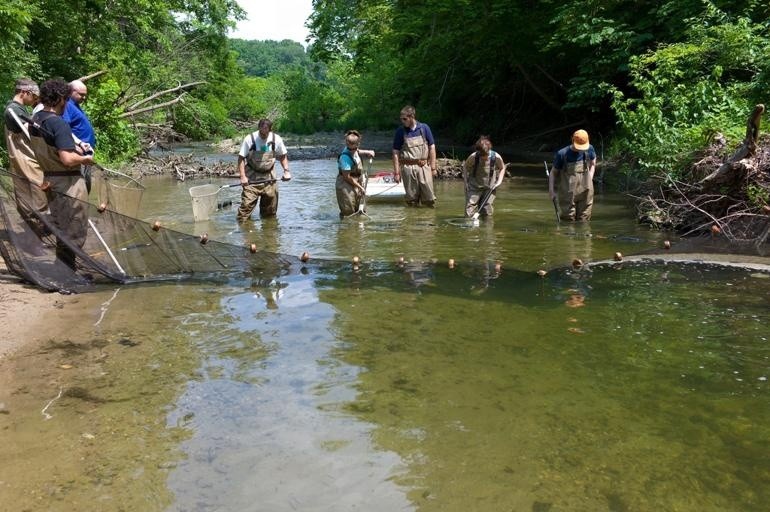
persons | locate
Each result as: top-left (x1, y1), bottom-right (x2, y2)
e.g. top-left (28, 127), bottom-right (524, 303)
top-left (462, 136), bottom-right (505, 219)
top-left (5, 77), bottom-right (52, 257)
top-left (31, 97), bottom-right (94, 155)
top-left (27, 80), bottom-right (95, 277)
top-left (59, 78), bottom-right (95, 195)
top-left (392, 105), bottom-right (438, 206)
top-left (549, 129), bottom-right (596, 222)
top-left (335, 130), bottom-right (376, 219)
top-left (235, 119), bottom-right (291, 224)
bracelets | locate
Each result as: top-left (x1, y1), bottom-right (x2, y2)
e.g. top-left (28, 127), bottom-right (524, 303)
top-left (79, 141), bottom-right (82, 147)
top-left (283, 168), bottom-right (290, 172)
top-left (393, 173), bottom-right (401, 175)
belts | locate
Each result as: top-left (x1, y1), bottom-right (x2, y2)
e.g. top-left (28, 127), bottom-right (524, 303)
top-left (247, 162), bottom-right (275, 174)
top-left (398, 158), bottom-right (428, 166)
top-left (44, 170), bottom-right (81, 177)
top-left (339, 171), bottom-right (361, 177)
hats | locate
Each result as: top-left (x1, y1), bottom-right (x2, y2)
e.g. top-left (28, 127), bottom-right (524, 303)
top-left (15, 78), bottom-right (40, 97)
top-left (573, 128), bottom-right (590, 151)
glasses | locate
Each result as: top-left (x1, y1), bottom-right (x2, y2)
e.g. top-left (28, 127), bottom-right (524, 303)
top-left (348, 131), bottom-right (360, 137)
top-left (400, 118), bottom-right (407, 120)
